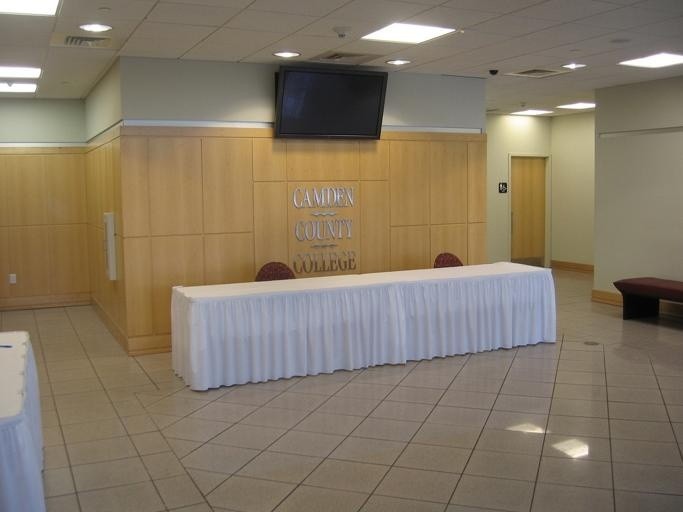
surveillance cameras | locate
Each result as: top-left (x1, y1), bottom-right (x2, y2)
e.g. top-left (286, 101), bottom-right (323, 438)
top-left (489, 68), bottom-right (499, 75)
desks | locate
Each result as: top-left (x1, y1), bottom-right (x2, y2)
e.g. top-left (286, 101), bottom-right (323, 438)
top-left (169, 262), bottom-right (557, 391)
top-left (0, 329), bottom-right (45, 510)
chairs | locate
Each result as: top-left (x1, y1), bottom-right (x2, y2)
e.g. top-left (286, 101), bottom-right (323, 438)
top-left (254, 262), bottom-right (295, 282)
top-left (434, 253), bottom-right (464, 268)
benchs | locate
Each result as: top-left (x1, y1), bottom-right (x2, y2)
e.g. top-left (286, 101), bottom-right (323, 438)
top-left (612, 275), bottom-right (683, 321)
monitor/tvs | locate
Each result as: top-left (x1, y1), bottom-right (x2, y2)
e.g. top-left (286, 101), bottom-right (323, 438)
top-left (276, 65), bottom-right (388, 140)
